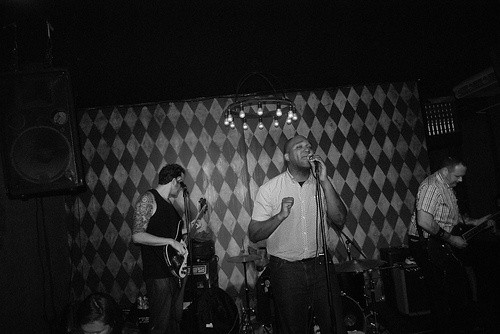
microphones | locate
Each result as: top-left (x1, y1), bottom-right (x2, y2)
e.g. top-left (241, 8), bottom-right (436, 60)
top-left (307, 154), bottom-right (323, 168)
top-left (177, 176), bottom-right (187, 191)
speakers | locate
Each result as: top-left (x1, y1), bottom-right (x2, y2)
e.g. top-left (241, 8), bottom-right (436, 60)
top-left (0, 67), bottom-right (87, 200)
top-left (393, 264), bottom-right (431, 316)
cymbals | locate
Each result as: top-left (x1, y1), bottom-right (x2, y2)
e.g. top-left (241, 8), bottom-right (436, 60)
top-left (328, 259), bottom-right (388, 272)
top-left (224, 255), bottom-right (262, 263)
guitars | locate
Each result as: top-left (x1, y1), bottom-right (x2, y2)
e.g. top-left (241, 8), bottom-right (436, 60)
top-left (162, 198), bottom-right (208, 280)
top-left (426, 211), bottom-right (500, 276)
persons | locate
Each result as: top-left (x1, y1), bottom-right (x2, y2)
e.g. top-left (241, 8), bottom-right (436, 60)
top-left (249, 136), bottom-right (348, 334)
top-left (407, 156), bottom-right (496, 334)
top-left (131, 164), bottom-right (205, 334)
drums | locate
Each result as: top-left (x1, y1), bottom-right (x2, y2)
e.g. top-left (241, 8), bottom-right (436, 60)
top-left (337, 289), bottom-right (374, 334)
top-left (218, 289), bottom-right (241, 334)
